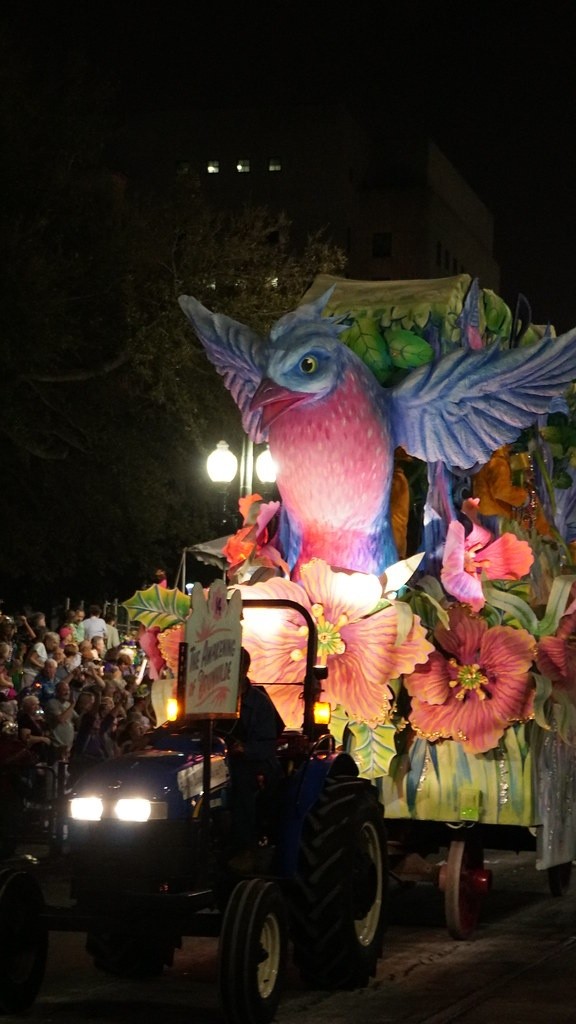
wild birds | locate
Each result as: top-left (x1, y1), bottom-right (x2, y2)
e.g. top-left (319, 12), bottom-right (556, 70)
top-left (179, 292), bottom-right (576, 599)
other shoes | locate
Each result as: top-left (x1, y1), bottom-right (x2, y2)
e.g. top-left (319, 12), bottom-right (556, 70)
top-left (227, 850), bottom-right (263, 874)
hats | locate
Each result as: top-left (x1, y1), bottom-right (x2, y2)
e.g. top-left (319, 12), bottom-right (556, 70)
top-left (84, 650), bottom-right (103, 661)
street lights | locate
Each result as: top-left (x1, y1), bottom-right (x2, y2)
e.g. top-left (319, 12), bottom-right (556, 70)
top-left (205, 431), bottom-right (279, 530)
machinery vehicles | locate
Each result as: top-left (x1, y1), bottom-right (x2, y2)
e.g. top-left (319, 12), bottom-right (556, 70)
top-left (1, 601), bottom-right (575, 1024)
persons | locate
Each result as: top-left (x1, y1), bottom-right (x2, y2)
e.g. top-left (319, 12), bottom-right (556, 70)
top-left (211, 646), bottom-right (286, 852)
top-left (154, 568), bottom-right (168, 588)
top-left (0, 603), bottom-right (158, 778)
